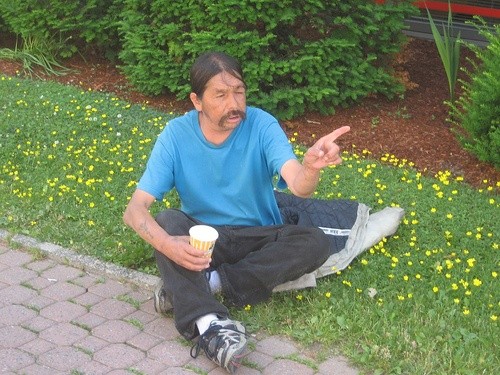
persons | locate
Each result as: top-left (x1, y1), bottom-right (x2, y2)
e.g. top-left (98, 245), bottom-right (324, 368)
top-left (121, 51), bottom-right (351, 375)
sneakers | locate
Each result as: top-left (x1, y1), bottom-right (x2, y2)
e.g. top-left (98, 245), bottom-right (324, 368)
top-left (153, 276), bottom-right (174, 314)
top-left (190, 318), bottom-right (249, 374)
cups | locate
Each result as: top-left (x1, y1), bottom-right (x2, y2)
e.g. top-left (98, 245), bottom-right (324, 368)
top-left (189, 224), bottom-right (219, 266)
